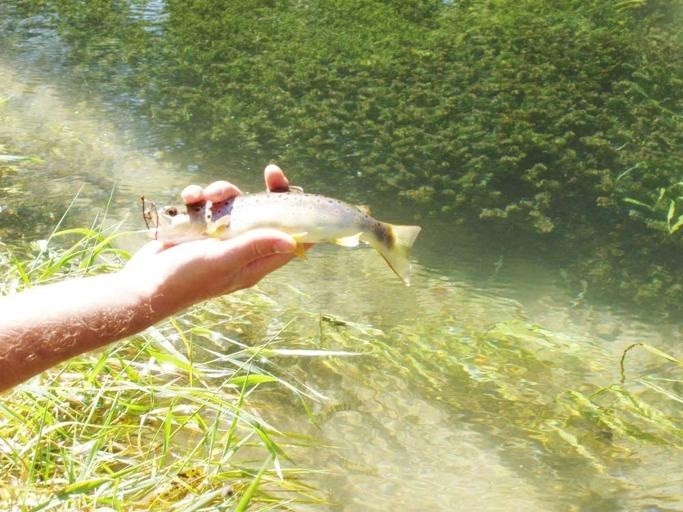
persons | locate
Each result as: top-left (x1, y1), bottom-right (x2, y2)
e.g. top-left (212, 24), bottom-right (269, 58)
top-left (0, 161), bottom-right (314, 403)
